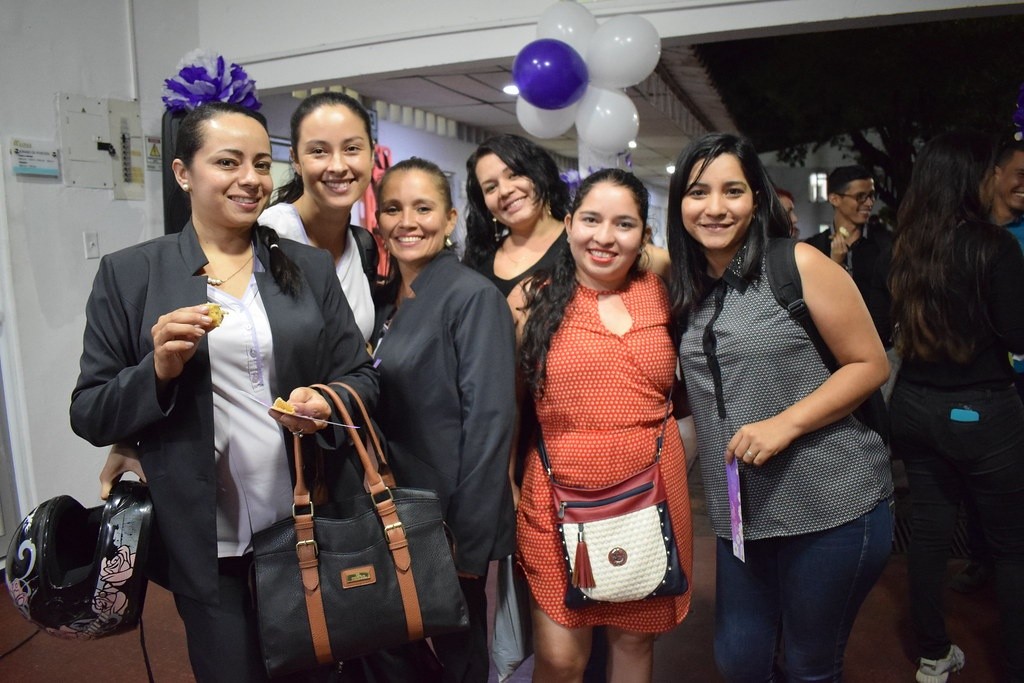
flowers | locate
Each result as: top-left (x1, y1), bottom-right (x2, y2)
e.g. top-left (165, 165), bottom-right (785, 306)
top-left (160, 47), bottom-right (264, 115)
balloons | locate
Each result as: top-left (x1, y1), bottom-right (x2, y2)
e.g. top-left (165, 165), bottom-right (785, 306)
top-left (512, 38), bottom-right (587, 107)
top-left (585, 14), bottom-right (661, 90)
top-left (537, 0), bottom-right (598, 57)
top-left (516, 93), bottom-right (577, 139)
top-left (576, 86), bottom-right (641, 155)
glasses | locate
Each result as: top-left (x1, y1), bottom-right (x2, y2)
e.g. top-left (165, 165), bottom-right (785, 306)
top-left (834, 190), bottom-right (878, 205)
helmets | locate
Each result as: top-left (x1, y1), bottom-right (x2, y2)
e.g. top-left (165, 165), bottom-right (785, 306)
top-left (6, 479), bottom-right (154, 643)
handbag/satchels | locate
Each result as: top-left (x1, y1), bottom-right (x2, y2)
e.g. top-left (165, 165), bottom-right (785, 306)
top-left (491, 554), bottom-right (535, 683)
top-left (250, 381), bottom-right (473, 671)
top-left (550, 464), bottom-right (688, 611)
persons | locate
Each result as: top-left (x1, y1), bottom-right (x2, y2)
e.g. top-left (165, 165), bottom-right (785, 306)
top-left (69, 102), bottom-right (391, 683)
top-left (886, 128), bottom-right (1024, 683)
top-left (663, 133), bottom-right (895, 683)
top-left (98, 92), bottom-right (382, 503)
top-left (368, 158), bottom-right (518, 683)
top-left (461, 135), bottom-right (672, 683)
top-left (501, 168), bottom-right (692, 683)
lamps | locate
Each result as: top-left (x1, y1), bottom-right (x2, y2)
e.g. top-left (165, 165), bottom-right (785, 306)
top-left (806, 168), bottom-right (829, 203)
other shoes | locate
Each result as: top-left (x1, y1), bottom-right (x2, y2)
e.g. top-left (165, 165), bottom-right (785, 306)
top-left (916, 645), bottom-right (964, 683)
top-left (947, 552), bottom-right (996, 594)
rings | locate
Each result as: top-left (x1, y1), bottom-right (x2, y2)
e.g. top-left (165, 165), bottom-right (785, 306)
top-left (747, 450), bottom-right (755, 458)
top-left (293, 429), bottom-right (303, 437)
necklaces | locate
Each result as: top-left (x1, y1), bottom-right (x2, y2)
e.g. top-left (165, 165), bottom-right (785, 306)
top-left (504, 222), bottom-right (558, 264)
top-left (204, 258), bottom-right (254, 286)
